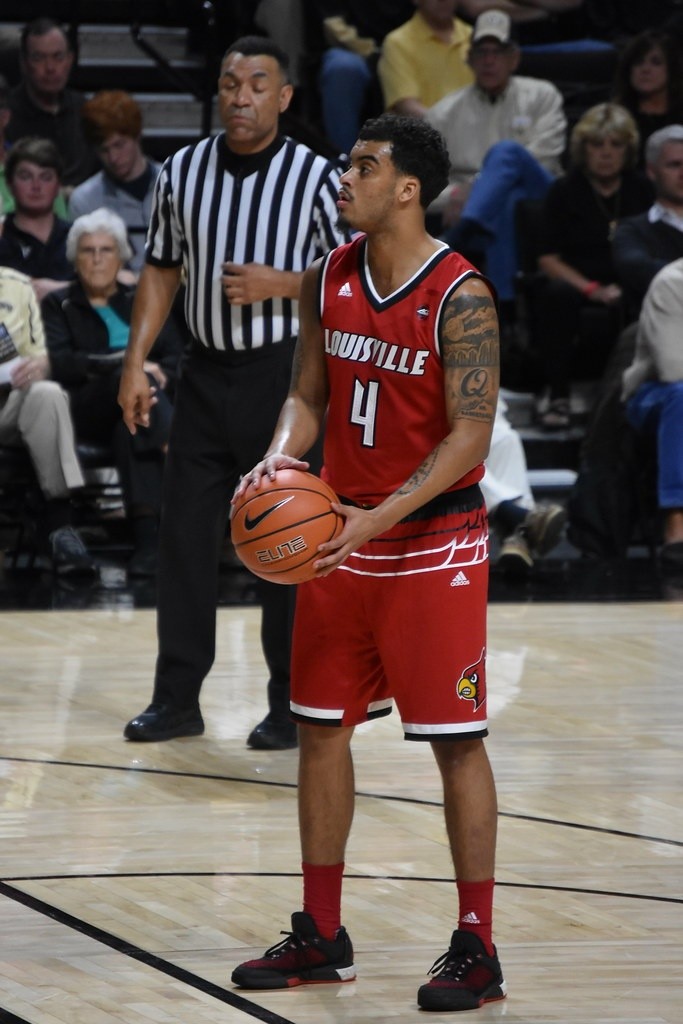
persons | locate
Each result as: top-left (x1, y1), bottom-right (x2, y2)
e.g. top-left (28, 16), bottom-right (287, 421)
top-left (117, 36), bottom-right (363, 751)
top-left (227, 110), bottom-right (506, 1013)
top-left (306, 0), bottom-right (683, 601)
top-left (1, 14), bottom-right (165, 300)
top-left (38, 205), bottom-right (190, 610)
top-left (0, 264), bottom-right (102, 583)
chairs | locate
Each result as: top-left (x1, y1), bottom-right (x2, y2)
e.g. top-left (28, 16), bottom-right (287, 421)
top-left (0, 193), bottom-right (669, 583)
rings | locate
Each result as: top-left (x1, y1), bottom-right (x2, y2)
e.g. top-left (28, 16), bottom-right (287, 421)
top-left (26, 373), bottom-right (32, 381)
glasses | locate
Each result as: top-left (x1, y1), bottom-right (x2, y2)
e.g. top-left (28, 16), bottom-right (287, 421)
top-left (472, 43), bottom-right (512, 58)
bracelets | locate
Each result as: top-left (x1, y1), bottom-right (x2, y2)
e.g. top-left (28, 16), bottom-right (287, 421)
top-left (584, 281), bottom-right (600, 295)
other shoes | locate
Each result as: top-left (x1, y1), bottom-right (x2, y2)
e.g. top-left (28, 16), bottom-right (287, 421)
top-left (496, 534), bottom-right (534, 583)
top-left (526, 504), bottom-right (573, 555)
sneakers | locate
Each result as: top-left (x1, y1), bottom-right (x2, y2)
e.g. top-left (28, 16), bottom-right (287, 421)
top-left (247, 713), bottom-right (298, 750)
top-left (232, 912), bottom-right (357, 989)
top-left (125, 702), bottom-right (205, 743)
top-left (50, 528), bottom-right (98, 578)
top-left (417, 931), bottom-right (507, 1011)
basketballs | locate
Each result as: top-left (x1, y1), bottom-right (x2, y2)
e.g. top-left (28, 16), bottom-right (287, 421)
top-left (230, 468), bottom-right (346, 585)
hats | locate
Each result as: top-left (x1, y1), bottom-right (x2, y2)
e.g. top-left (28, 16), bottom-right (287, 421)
top-left (468, 9), bottom-right (521, 48)
